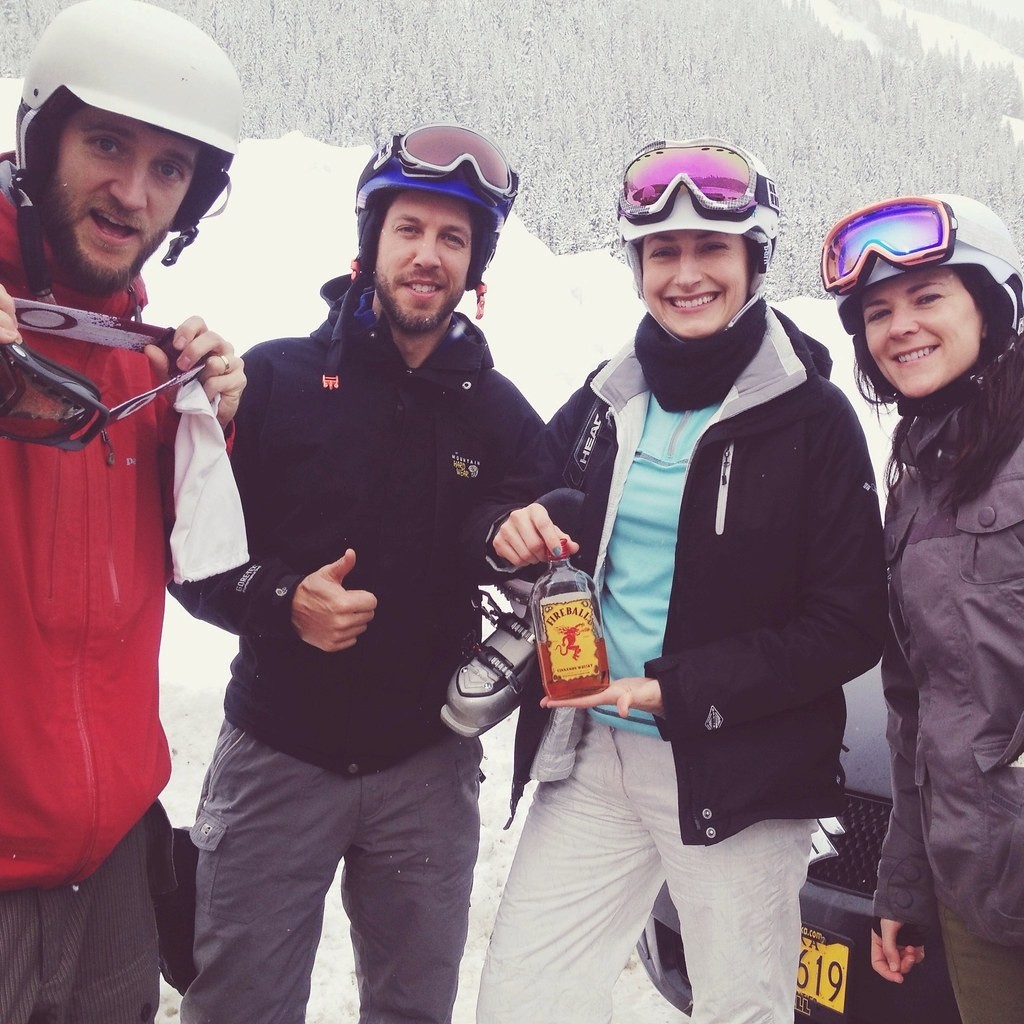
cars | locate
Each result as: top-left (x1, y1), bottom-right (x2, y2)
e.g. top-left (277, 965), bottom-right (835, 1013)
top-left (632, 648), bottom-right (962, 1024)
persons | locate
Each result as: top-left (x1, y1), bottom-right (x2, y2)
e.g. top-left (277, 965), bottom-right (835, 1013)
top-left (821, 195), bottom-right (1024, 1024)
top-left (0, 4), bottom-right (245, 1024)
top-left (463, 138), bottom-right (896, 1024)
top-left (158, 127), bottom-right (563, 1024)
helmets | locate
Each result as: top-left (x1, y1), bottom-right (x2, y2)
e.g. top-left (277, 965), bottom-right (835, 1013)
top-left (616, 140), bottom-right (777, 296)
top-left (358, 139), bottom-right (511, 290)
top-left (836, 196), bottom-right (1022, 363)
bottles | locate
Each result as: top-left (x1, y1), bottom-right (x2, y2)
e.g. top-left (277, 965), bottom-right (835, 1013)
top-left (528, 540), bottom-right (611, 699)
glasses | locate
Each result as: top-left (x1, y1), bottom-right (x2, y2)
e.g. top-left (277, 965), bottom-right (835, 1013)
top-left (819, 198), bottom-right (1022, 293)
top-left (616, 139), bottom-right (781, 222)
top-left (0, 298), bottom-right (204, 451)
top-left (355, 126), bottom-right (518, 223)
top-left (14, 2), bottom-right (244, 230)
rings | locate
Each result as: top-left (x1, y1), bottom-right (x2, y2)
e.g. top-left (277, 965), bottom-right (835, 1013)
top-left (219, 353), bottom-right (230, 375)
